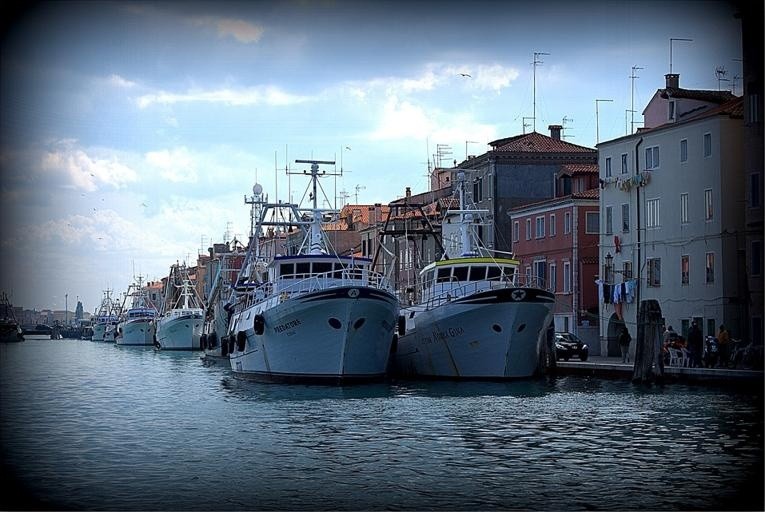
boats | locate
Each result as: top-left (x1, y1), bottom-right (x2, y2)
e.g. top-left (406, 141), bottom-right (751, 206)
top-left (0, 291), bottom-right (26, 342)
top-left (154, 258), bottom-right (208, 349)
top-left (225, 144), bottom-right (402, 387)
top-left (113, 258), bottom-right (158, 347)
top-left (51, 289), bottom-right (123, 342)
top-left (200, 253), bottom-right (231, 358)
top-left (392, 170), bottom-right (558, 382)
top-left (24, 323), bottom-right (51, 336)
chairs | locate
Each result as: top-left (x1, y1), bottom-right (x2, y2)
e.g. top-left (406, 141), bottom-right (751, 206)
top-left (668, 345), bottom-right (694, 367)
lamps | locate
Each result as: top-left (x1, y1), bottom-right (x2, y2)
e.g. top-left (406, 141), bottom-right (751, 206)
top-left (605, 251), bottom-right (632, 281)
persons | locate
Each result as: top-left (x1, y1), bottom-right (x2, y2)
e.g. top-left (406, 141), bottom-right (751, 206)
top-left (663, 321), bottom-right (730, 369)
top-left (619, 327), bottom-right (632, 363)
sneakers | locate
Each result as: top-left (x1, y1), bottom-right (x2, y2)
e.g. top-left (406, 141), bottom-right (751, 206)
top-left (622, 358), bottom-right (630, 364)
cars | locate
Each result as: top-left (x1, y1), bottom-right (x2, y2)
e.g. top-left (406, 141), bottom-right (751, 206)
top-left (554, 331), bottom-right (586, 361)
